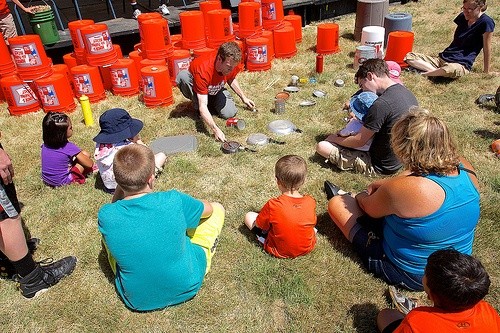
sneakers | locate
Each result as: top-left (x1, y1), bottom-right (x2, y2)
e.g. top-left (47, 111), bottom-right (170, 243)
top-left (0, 237), bottom-right (40, 278)
top-left (17, 256), bottom-right (78, 298)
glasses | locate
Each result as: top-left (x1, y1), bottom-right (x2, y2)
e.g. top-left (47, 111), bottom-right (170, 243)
top-left (460, 4), bottom-right (478, 14)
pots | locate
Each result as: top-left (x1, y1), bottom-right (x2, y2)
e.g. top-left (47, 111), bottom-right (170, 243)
top-left (266, 119), bottom-right (304, 135)
top-left (246, 132), bottom-right (286, 146)
top-left (220, 141), bottom-right (258, 154)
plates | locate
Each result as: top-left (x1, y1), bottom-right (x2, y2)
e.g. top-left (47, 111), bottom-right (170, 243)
top-left (275, 92), bottom-right (289, 100)
top-left (313, 88), bottom-right (327, 98)
top-left (283, 86), bottom-right (301, 92)
top-left (299, 100), bottom-right (316, 106)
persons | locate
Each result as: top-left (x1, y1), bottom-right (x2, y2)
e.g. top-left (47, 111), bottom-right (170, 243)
top-left (96, 144), bottom-right (225, 313)
top-left (243, 156), bottom-right (318, 258)
top-left (315, 61), bottom-right (420, 177)
top-left (92, 109), bottom-right (167, 190)
top-left (0, 139), bottom-right (77, 301)
top-left (0, 0), bottom-right (35, 47)
top-left (326, 91), bottom-right (379, 151)
top-left (40, 111), bottom-right (96, 192)
top-left (376, 247), bottom-right (500, 333)
top-left (327, 113), bottom-right (482, 291)
top-left (129, 0), bottom-right (170, 19)
top-left (176, 41), bottom-right (256, 144)
top-left (404, 0), bottom-right (497, 80)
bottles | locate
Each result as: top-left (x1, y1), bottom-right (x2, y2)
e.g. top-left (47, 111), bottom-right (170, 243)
top-left (316, 53), bottom-right (323, 73)
top-left (290, 76), bottom-right (298, 86)
top-left (299, 78), bottom-right (316, 83)
top-left (79, 94), bottom-right (94, 125)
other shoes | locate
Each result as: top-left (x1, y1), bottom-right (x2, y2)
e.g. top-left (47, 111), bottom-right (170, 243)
top-left (388, 285), bottom-right (418, 315)
top-left (324, 179), bottom-right (352, 200)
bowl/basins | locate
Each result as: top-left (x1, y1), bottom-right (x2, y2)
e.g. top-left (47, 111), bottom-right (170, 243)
top-left (334, 79), bottom-right (345, 87)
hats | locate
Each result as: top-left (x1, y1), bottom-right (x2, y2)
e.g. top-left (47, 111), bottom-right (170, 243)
top-left (384, 61), bottom-right (402, 84)
top-left (92, 108), bottom-right (144, 144)
top-left (349, 91), bottom-right (379, 121)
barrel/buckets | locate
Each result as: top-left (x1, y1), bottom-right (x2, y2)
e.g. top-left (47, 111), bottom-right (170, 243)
top-left (361, 25), bottom-right (385, 60)
top-left (0, 0), bottom-right (303, 109)
top-left (227, 118), bottom-right (246, 130)
top-left (384, 12), bottom-right (413, 48)
top-left (315, 23), bottom-right (341, 55)
top-left (274, 99), bottom-right (285, 113)
top-left (353, 44), bottom-right (382, 70)
top-left (352, 0), bottom-right (390, 42)
top-left (384, 31), bottom-right (414, 69)
